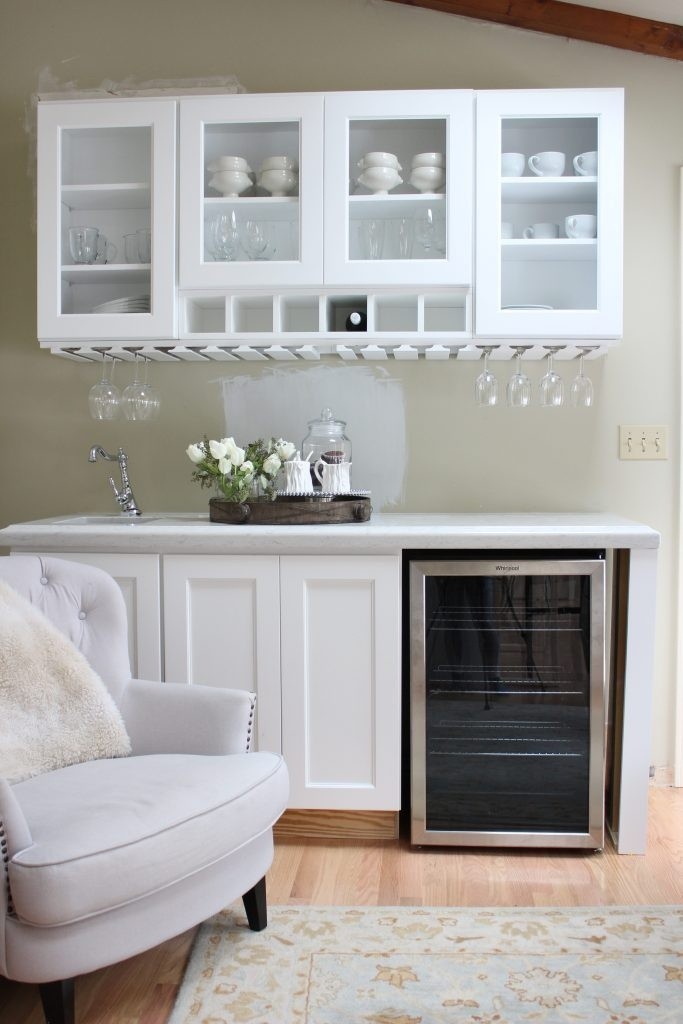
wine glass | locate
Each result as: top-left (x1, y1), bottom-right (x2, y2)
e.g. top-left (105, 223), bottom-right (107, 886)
top-left (506, 346), bottom-right (532, 406)
top-left (540, 353), bottom-right (565, 407)
top-left (572, 353), bottom-right (593, 407)
top-left (414, 208), bottom-right (439, 259)
top-left (89, 353), bottom-right (160, 420)
top-left (474, 347), bottom-right (499, 406)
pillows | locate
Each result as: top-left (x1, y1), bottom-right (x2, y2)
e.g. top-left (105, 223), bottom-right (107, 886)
top-left (0, 583), bottom-right (132, 785)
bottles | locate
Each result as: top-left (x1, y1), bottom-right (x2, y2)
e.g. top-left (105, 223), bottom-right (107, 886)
top-left (344, 309), bottom-right (368, 332)
top-left (301, 408), bottom-right (352, 493)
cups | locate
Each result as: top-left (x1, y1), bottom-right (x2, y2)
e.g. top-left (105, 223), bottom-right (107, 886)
top-left (256, 155), bottom-right (298, 197)
top-left (207, 208), bottom-right (277, 261)
top-left (565, 214), bottom-right (597, 237)
top-left (314, 460), bottom-right (352, 495)
top-left (364, 218), bottom-right (411, 259)
top-left (501, 222), bottom-right (518, 239)
top-left (523, 223), bottom-right (559, 238)
top-left (410, 153), bottom-right (446, 193)
top-left (502, 152), bottom-right (525, 177)
top-left (278, 460), bottom-right (314, 493)
top-left (207, 156), bottom-right (253, 197)
top-left (528, 151), bottom-right (566, 177)
top-left (357, 152), bottom-right (405, 195)
top-left (68, 226), bottom-right (152, 264)
top-left (573, 151), bottom-right (598, 175)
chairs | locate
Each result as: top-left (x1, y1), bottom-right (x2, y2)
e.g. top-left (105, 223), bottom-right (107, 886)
top-left (0, 554), bottom-right (287, 1024)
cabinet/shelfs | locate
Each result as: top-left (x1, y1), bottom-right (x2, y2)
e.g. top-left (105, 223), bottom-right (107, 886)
top-left (37, 88), bottom-right (625, 349)
top-left (9, 551), bottom-right (402, 811)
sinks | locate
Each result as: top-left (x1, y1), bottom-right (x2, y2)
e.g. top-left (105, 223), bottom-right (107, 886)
top-left (52, 517), bottom-right (163, 526)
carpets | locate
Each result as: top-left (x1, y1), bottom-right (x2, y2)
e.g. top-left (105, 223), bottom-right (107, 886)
top-left (168, 902), bottom-right (683, 1024)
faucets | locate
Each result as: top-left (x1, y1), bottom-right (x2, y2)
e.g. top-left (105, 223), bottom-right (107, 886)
top-left (88, 445), bottom-right (142, 515)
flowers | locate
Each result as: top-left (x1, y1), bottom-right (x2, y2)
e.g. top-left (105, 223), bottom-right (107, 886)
top-left (185, 434), bottom-right (296, 502)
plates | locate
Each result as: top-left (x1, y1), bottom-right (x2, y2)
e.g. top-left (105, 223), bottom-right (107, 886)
top-left (91, 293), bottom-right (149, 314)
top-left (502, 304), bottom-right (551, 309)
